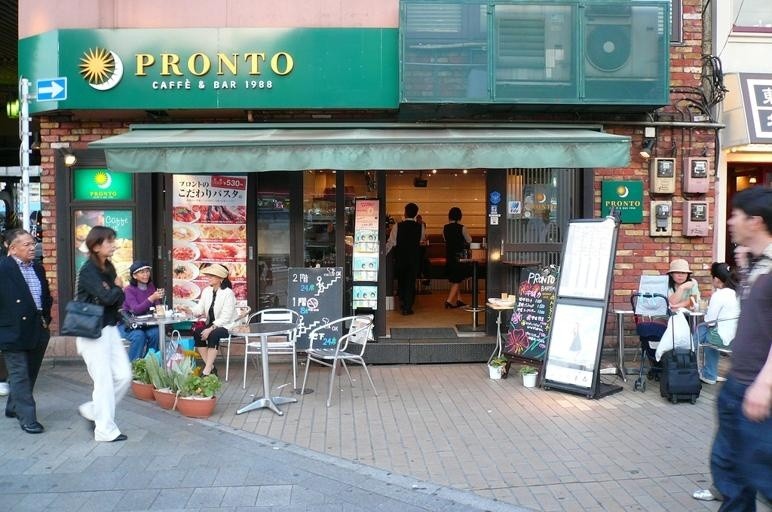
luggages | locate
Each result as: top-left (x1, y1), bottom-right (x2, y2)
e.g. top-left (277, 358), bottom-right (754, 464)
top-left (660, 312), bottom-right (702, 404)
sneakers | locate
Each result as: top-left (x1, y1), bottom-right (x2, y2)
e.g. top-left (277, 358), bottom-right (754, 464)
top-left (698, 368), bottom-right (716, 385)
top-left (693, 490), bottom-right (715, 501)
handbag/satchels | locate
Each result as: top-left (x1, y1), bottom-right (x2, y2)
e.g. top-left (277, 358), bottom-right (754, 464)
top-left (165, 330), bottom-right (197, 369)
top-left (61, 300), bottom-right (105, 338)
top-left (121, 307), bottom-right (159, 332)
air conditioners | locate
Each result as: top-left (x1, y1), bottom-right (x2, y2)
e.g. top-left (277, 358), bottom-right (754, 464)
top-left (581, 6), bottom-right (659, 81)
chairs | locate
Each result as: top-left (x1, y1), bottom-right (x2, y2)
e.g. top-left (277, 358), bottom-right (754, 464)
top-left (697, 315), bottom-right (739, 381)
top-left (300, 314), bottom-right (378, 407)
top-left (120, 338), bottom-right (132, 348)
top-left (218, 306), bottom-right (251, 381)
top-left (243, 308), bottom-right (300, 389)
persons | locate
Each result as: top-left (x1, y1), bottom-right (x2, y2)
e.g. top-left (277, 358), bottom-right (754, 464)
top-left (654, 259), bottom-right (701, 322)
top-left (172, 263), bottom-right (238, 380)
top-left (692, 484), bottom-right (723, 501)
top-left (116, 258), bottom-right (166, 363)
top-left (76, 225), bottom-right (134, 443)
top-left (710, 188), bottom-right (772, 512)
top-left (442, 206), bottom-right (473, 308)
top-left (694, 262), bottom-right (740, 384)
top-left (0, 209), bottom-right (53, 434)
top-left (381, 203), bottom-right (426, 316)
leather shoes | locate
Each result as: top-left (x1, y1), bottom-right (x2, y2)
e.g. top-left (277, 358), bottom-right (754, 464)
top-left (21, 422), bottom-right (43, 433)
top-left (113, 434), bottom-right (127, 441)
top-left (200, 367), bottom-right (217, 377)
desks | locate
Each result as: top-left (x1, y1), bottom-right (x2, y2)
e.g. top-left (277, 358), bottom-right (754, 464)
top-left (228, 322), bottom-right (298, 416)
top-left (454, 258), bottom-right (542, 338)
top-left (486, 303), bottom-right (515, 365)
top-left (132, 313), bottom-right (192, 363)
top-left (600, 309), bottom-right (705, 383)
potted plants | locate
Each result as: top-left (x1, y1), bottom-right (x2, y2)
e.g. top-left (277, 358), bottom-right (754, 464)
top-left (176, 374), bottom-right (222, 418)
top-left (519, 365), bottom-right (539, 387)
top-left (131, 355), bottom-right (154, 400)
top-left (153, 370), bottom-right (178, 410)
top-left (488, 356), bottom-right (507, 379)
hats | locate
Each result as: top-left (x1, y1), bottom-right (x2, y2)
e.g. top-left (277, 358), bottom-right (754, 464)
top-left (130, 261), bottom-right (152, 273)
top-left (200, 264), bottom-right (229, 278)
top-left (666, 259), bottom-right (693, 273)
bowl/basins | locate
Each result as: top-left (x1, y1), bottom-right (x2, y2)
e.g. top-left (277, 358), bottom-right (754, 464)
top-left (495, 300), bottom-right (513, 307)
top-left (469, 242), bottom-right (481, 249)
top-left (488, 297), bottom-right (500, 304)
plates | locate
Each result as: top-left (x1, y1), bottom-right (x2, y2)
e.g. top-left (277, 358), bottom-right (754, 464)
top-left (173, 204), bottom-right (201, 305)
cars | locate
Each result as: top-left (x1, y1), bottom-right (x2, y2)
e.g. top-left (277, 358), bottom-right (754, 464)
top-left (305, 221), bottom-right (336, 252)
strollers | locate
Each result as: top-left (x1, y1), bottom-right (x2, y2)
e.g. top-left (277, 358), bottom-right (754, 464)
top-left (630, 292), bottom-right (681, 394)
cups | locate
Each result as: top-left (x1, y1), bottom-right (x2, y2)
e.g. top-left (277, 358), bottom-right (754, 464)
top-left (156, 304), bottom-right (166, 318)
top-left (689, 294), bottom-right (697, 311)
top-left (508, 295), bottom-right (515, 302)
top-left (501, 292), bottom-right (507, 300)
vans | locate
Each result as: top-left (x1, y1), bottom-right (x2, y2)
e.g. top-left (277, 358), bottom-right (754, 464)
top-left (257, 207), bottom-right (290, 238)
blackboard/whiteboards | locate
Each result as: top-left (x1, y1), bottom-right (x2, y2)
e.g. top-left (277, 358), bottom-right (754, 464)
top-left (287, 266), bottom-right (343, 354)
top-left (501, 265), bottom-right (561, 362)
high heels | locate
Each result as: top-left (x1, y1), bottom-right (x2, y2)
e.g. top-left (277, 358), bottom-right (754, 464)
top-left (457, 300), bottom-right (466, 307)
top-left (445, 302), bottom-right (458, 308)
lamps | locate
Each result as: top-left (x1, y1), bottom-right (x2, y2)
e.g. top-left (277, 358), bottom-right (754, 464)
top-left (31, 142), bottom-right (77, 167)
top-left (639, 140), bottom-right (657, 158)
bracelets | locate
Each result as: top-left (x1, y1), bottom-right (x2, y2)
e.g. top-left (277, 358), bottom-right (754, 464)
top-left (211, 325), bottom-right (214, 329)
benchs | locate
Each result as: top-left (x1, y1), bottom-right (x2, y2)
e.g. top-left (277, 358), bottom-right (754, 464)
top-left (425, 233), bottom-right (486, 292)
